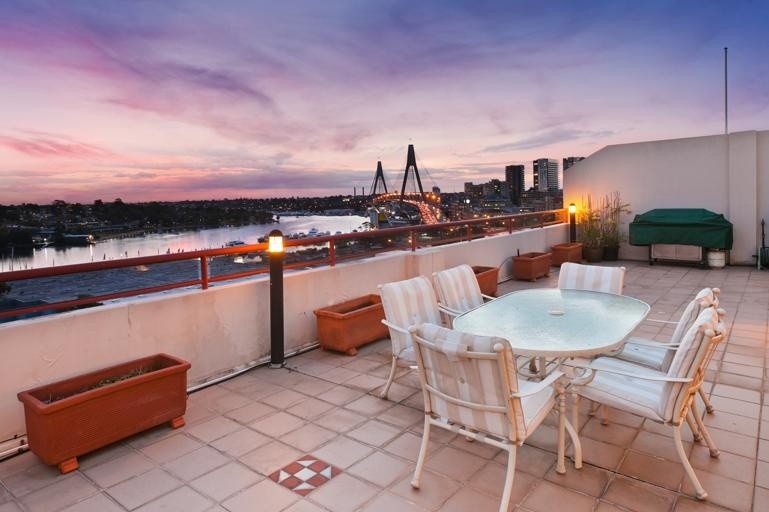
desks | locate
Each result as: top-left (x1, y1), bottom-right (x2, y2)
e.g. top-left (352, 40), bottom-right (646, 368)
top-left (451, 287), bottom-right (652, 475)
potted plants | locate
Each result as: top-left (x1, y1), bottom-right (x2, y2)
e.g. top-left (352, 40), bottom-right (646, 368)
top-left (580, 191), bottom-right (633, 262)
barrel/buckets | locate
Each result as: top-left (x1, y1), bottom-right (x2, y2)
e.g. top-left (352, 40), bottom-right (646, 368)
top-left (708, 250), bottom-right (725, 268)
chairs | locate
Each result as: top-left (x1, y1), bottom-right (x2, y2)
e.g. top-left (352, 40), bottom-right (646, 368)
top-left (406, 321), bottom-right (568, 511)
top-left (378, 273), bottom-right (446, 400)
top-left (432, 263), bottom-right (496, 330)
top-left (588, 285), bottom-right (726, 443)
top-left (569, 307), bottom-right (723, 501)
top-left (558, 262), bottom-right (626, 418)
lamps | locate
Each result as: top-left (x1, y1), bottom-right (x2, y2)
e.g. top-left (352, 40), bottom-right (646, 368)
top-left (569, 204), bottom-right (575, 213)
top-left (265, 230), bottom-right (286, 257)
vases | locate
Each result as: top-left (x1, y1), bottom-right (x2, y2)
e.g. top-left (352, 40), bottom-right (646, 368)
top-left (513, 252), bottom-right (555, 281)
top-left (553, 242), bottom-right (583, 266)
top-left (316, 293), bottom-right (388, 356)
top-left (16, 351), bottom-right (193, 475)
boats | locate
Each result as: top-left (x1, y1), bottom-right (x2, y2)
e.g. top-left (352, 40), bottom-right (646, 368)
top-left (287, 229), bottom-right (331, 239)
top-left (227, 241), bottom-right (244, 246)
